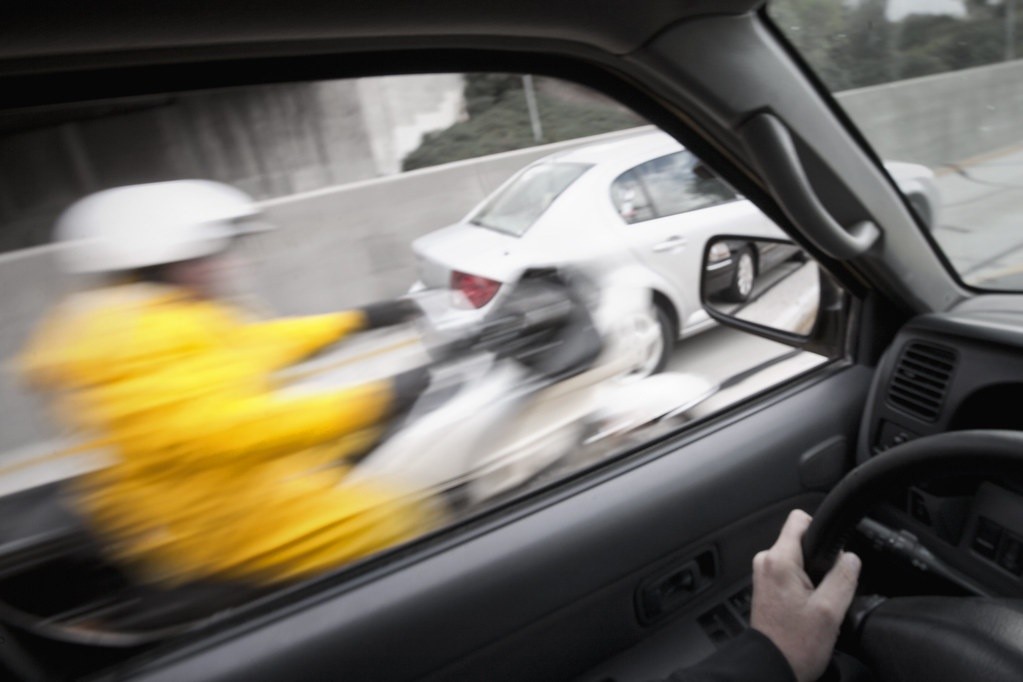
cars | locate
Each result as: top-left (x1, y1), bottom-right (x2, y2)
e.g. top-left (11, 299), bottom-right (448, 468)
top-left (405, 129), bottom-right (939, 380)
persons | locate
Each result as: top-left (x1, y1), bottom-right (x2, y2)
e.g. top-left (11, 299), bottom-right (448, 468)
top-left (657, 508), bottom-right (861, 682)
top-left (8, 178), bottom-right (529, 585)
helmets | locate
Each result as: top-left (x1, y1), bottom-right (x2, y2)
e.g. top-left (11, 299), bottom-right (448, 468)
top-left (53, 177), bottom-right (254, 273)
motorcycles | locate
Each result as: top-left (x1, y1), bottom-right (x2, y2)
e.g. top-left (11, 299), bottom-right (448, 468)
top-left (0, 265), bottom-right (725, 638)
